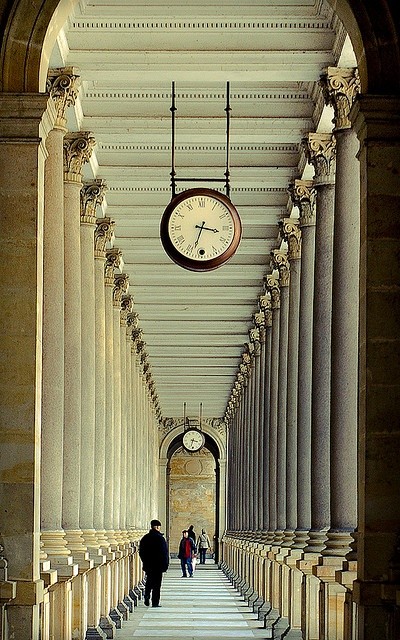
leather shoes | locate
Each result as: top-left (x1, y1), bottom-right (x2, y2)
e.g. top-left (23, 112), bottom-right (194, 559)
top-left (152, 604), bottom-right (162, 607)
top-left (144, 596), bottom-right (149, 606)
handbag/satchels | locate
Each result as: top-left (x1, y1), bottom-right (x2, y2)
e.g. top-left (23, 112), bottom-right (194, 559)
top-left (202, 535), bottom-right (210, 547)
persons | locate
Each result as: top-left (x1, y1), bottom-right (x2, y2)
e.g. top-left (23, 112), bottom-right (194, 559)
top-left (197, 528), bottom-right (211, 564)
top-left (187, 525), bottom-right (196, 543)
top-left (177, 530), bottom-right (195, 578)
top-left (139, 520), bottom-right (169, 609)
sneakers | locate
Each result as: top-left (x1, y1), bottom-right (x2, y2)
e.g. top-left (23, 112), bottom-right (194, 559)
top-left (203, 562), bottom-right (205, 564)
top-left (181, 575), bottom-right (187, 578)
top-left (189, 575), bottom-right (193, 578)
top-left (199, 563), bottom-right (203, 564)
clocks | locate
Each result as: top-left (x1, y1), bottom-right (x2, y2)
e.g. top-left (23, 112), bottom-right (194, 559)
top-left (161, 187), bottom-right (243, 272)
top-left (181, 428), bottom-right (205, 452)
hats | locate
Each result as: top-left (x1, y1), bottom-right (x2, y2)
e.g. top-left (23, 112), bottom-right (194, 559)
top-left (182, 529), bottom-right (188, 533)
top-left (151, 520), bottom-right (161, 526)
